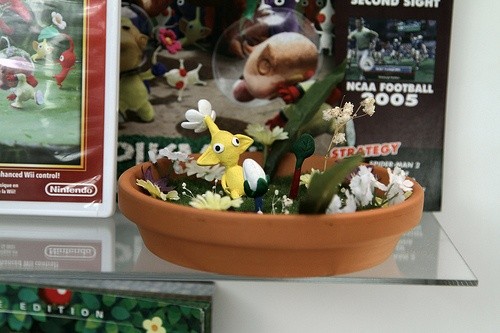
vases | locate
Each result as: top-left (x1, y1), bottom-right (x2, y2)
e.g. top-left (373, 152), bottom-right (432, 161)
top-left (118, 152), bottom-right (424, 278)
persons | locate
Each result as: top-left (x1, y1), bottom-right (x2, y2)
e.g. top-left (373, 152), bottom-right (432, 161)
top-left (347, 17), bottom-right (379, 82)
top-left (347, 29), bottom-right (435, 74)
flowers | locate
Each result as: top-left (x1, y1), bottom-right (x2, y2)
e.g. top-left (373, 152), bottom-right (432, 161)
top-left (135, 29), bottom-right (415, 216)
